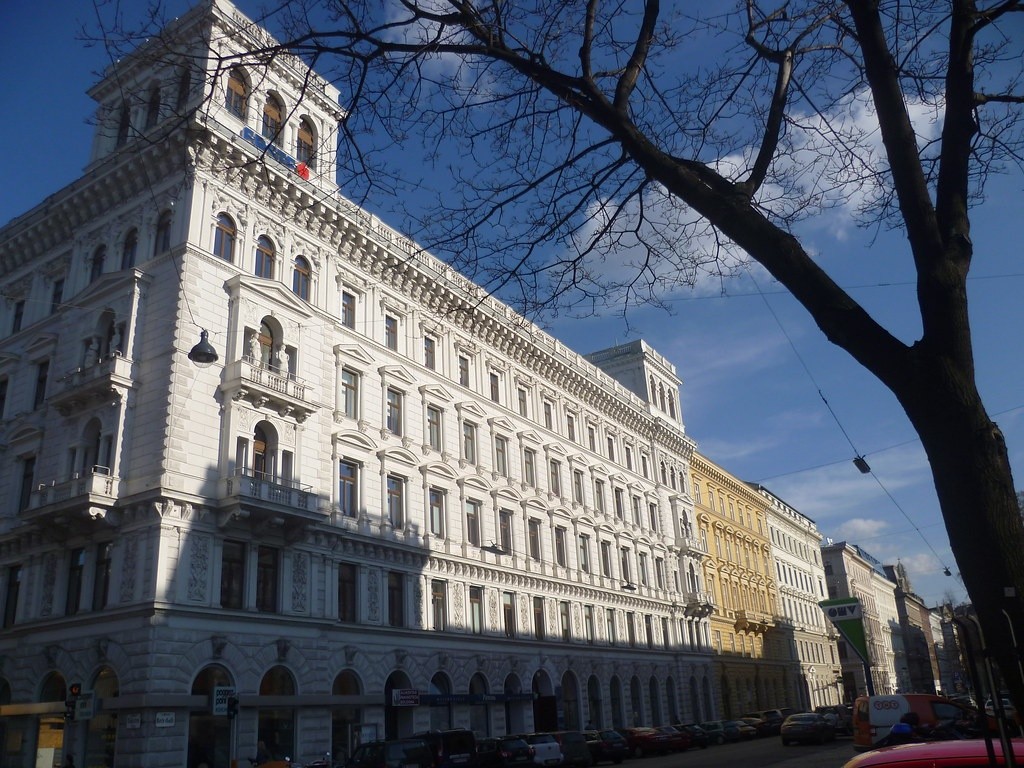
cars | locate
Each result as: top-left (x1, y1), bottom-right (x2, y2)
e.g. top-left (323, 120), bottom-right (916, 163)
top-left (334, 694), bottom-right (1024, 768)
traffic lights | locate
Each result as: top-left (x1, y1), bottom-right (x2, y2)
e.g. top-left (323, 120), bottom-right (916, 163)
top-left (227, 697), bottom-right (238, 719)
top-left (63, 682), bottom-right (81, 721)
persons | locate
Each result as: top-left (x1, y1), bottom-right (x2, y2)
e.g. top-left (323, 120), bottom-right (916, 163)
top-left (585, 720), bottom-right (595, 730)
top-left (62, 754), bottom-right (76, 768)
top-left (256, 741), bottom-right (272, 765)
top-left (848, 691), bottom-right (857, 702)
top-left (673, 715), bottom-right (681, 724)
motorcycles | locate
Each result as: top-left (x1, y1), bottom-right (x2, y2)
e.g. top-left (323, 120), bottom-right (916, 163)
top-left (246, 751), bottom-right (332, 768)
